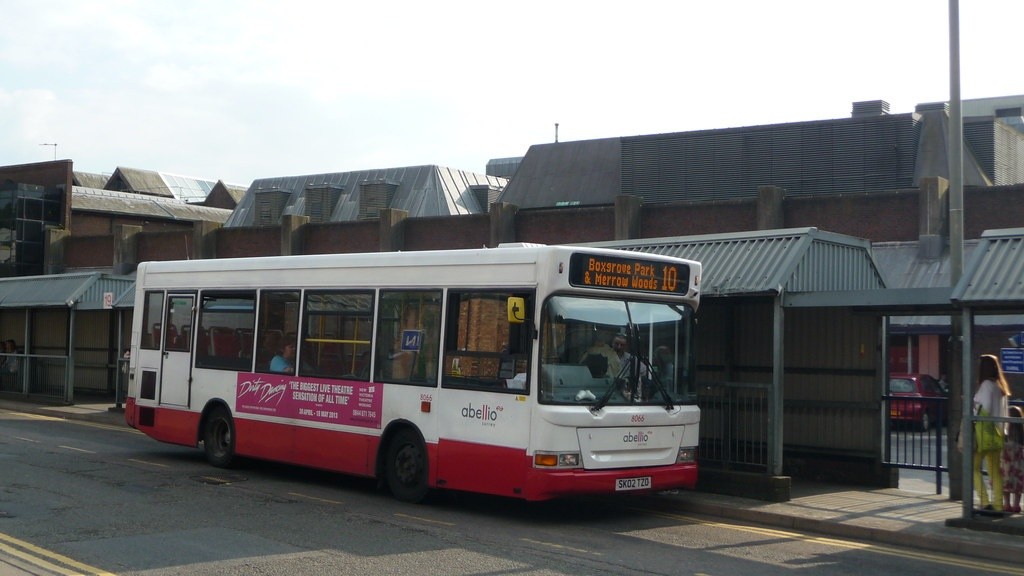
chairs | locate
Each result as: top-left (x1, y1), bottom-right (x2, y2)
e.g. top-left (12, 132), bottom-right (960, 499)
top-left (181, 324), bottom-right (206, 351)
top-left (151, 323), bottom-right (180, 350)
top-left (311, 332), bottom-right (342, 372)
top-left (208, 324), bottom-right (241, 361)
top-left (259, 328), bottom-right (282, 355)
top-left (236, 327), bottom-right (255, 356)
top-left (287, 332), bottom-right (312, 359)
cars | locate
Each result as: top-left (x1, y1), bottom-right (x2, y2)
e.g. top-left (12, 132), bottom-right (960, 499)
top-left (889, 374), bottom-right (948, 432)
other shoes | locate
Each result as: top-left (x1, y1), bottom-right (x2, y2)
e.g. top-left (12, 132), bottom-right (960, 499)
top-left (1003, 505), bottom-right (1012, 513)
top-left (972, 504), bottom-right (993, 515)
top-left (1012, 507), bottom-right (1021, 513)
top-left (985, 510), bottom-right (1003, 519)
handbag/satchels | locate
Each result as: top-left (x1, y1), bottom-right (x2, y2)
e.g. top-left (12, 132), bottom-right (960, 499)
top-left (957, 420), bottom-right (977, 455)
top-left (975, 406), bottom-right (1004, 453)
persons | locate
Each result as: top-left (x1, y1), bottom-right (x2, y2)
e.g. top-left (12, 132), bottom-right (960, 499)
top-left (578, 325), bottom-right (673, 403)
top-left (957, 354), bottom-right (1024, 512)
top-left (0, 339), bottom-right (21, 372)
top-left (269, 336), bottom-right (316, 373)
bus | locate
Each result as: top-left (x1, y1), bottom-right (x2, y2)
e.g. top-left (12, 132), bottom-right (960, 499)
top-left (123, 242), bottom-right (702, 507)
